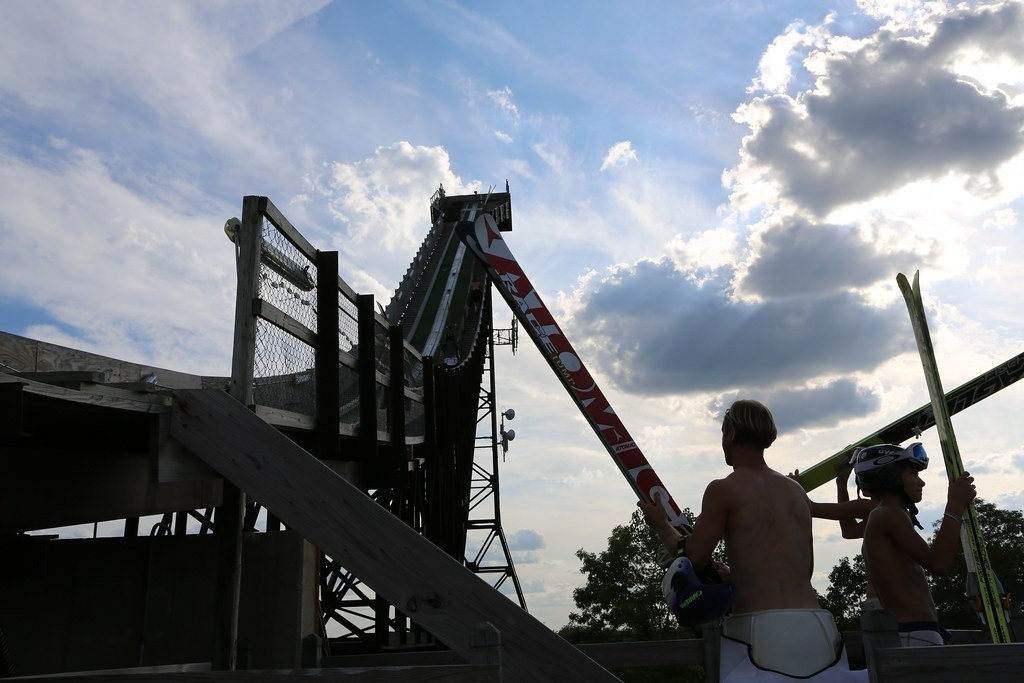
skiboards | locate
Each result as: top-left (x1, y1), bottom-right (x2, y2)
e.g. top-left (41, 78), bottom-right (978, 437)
top-left (454, 212), bottom-right (693, 540)
top-left (896, 269), bottom-right (1013, 644)
top-left (789, 351), bottom-right (1024, 493)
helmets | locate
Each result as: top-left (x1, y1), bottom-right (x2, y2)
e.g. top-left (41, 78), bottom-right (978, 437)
top-left (854, 443), bottom-right (928, 473)
top-left (659, 557), bottom-right (733, 628)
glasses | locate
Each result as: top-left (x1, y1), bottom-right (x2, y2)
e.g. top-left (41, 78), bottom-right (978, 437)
top-left (906, 443), bottom-right (928, 469)
top-left (849, 447), bottom-right (861, 465)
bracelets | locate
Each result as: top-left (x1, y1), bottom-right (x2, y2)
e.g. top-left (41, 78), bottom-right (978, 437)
top-left (944, 510), bottom-right (963, 524)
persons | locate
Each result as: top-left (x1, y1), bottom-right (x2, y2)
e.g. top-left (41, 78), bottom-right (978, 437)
top-left (855, 444), bottom-right (977, 647)
top-left (786, 443), bottom-right (915, 539)
top-left (637, 400), bottom-right (839, 682)
